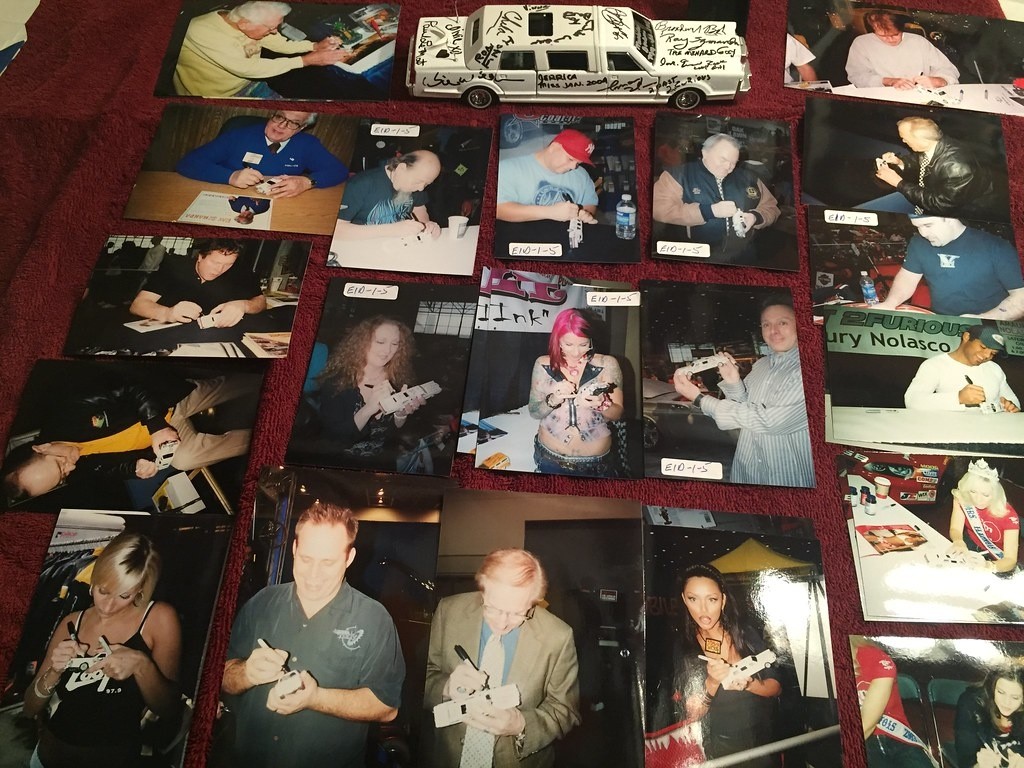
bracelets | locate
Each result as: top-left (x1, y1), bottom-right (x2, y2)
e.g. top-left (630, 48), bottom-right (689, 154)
top-left (42, 665), bottom-right (61, 693)
top-left (593, 394), bottom-right (611, 412)
top-left (394, 413), bottom-right (407, 419)
top-left (584, 209), bottom-right (593, 216)
top-left (692, 393), bottom-right (704, 409)
top-left (695, 680), bottom-right (714, 707)
top-left (34, 676), bottom-right (55, 699)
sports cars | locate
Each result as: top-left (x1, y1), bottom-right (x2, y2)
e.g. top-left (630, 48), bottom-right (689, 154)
top-left (642, 378), bottom-right (742, 454)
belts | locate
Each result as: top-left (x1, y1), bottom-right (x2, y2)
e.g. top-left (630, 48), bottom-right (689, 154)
top-left (536, 436), bottom-right (611, 462)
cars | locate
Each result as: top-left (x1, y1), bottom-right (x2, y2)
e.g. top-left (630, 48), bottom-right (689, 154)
top-left (833, 0), bottom-right (958, 64)
top-left (405, 4), bottom-right (753, 111)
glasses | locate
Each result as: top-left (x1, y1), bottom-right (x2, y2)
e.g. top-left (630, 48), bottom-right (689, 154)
top-left (262, 24), bottom-right (276, 32)
top-left (481, 596), bottom-right (536, 622)
top-left (271, 115), bottom-right (305, 130)
top-left (43, 454), bottom-right (68, 486)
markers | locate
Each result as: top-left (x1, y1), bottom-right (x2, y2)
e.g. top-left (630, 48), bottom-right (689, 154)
top-left (135, 457), bottom-right (157, 473)
top-left (99, 636), bottom-right (113, 656)
top-left (453, 643), bottom-right (491, 693)
top-left (257, 637), bottom-right (291, 674)
top-left (242, 161), bottom-right (265, 184)
top-left (713, 195), bottom-right (723, 201)
top-left (365, 384), bottom-right (396, 395)
top-left (201, 311), bottom-right (205, 316)
top-left (558, 373), bottom-right (576, 395)
top-left (404, 212), bottom-right (421, 241)
top-left (980, 550), bottom-right (990, 555)
top-left (326, 32), bottom-right (344, 48)
top-left (914, 71), bottom-right (924, 86)
top-left (562, 191), bottom-right (570, 202)
top-left (730, 359), bottom-right (747, 372)
top-left (698, 654), bottom-right (714, 662)
top-left (67, 620), bottom-right (86, 659)
top-left (977, 731), bottom-right (1004, 768)
top-left (883, 151), bottom-right (901, 164)
top-left (964, 374), bottom-right (973, 385)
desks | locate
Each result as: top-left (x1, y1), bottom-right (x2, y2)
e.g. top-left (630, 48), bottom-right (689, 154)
top-left (851, 475), bottom-right (1009, 622)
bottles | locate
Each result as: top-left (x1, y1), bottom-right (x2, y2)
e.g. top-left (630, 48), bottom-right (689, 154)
top-left (865, 494), bottom-right (877, 516)
top-left (860, 486), bottom-right (870, 505)
top-left (616, 194), bottom-right (636, 240)
top-left (850, 486), bottom-right (858, 506)
top-left (860, 271), bottom-right (879, 306)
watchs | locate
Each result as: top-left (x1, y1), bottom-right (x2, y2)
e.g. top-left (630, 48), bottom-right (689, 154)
top-left (302, 174), bottom-right (316, 189)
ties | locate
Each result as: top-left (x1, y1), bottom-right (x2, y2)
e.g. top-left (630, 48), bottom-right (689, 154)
top-left (915, 155), bottom-right (929, 215)
top-left (271, 142), bottom-right (279, 154)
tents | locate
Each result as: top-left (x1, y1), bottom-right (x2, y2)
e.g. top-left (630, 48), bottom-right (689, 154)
top-left (707, 538), bottom-right (817, 585)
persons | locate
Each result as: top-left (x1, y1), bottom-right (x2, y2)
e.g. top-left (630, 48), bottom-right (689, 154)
top-left (948, 457), bottom-right (1020, 573)
top-left (784, 9), bottom-right (961, 91)
top-left (868, 116), bottom-right (1024, 322)
top-left (653, 133), bottom-right (782, 243)
top-left (953, 671), bottom-right (1024, 768)
top-left (174, 109), bottom-right (350, 199)
top-left (903, 325), bottom-right (1021, 414)
top-left (849, 634), bottom-right (939, 768)
top-left (173, 1), bottom-right (353, 100)
top-left (0, 236), bottom-right (843, 768)
top-left (338, 150), bottom-right (441, 238)
top-left (497, 128), bottom-right (599, 225)
top-left (228, 195), bottom-right (270, 225)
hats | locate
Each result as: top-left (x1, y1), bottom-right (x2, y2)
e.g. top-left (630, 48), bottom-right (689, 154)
top-left (554, 129), bottom-right (596, 168)
top-left (460, 634), bottom-right (505, 768)
top-left (969, 325), bottom-right (1005, 351)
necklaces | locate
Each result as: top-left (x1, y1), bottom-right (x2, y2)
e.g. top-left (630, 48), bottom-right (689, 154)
top-left (696, 627), bottom-right (725, 654)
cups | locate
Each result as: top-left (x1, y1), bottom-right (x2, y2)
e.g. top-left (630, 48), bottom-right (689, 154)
top-left (874, 477), bottom-right (892, 499)
top-left (448, 215), bottom-right (469, 240)
